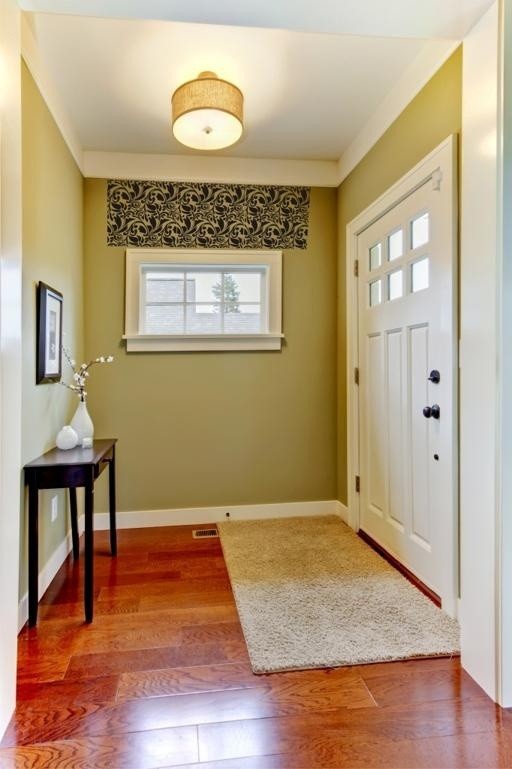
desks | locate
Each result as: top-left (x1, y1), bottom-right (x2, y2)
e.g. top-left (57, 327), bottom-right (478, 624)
top-left (26, 437), bottom-right (122, 634)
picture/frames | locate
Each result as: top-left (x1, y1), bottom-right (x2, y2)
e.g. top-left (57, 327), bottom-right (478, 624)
top-left (35, 277), bottom-right (63, 386)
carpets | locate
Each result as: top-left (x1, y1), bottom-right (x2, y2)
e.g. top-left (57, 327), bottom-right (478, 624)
top-left (213, 514), bottom-right (463, 679)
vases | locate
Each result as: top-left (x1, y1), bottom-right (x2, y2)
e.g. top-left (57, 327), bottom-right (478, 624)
top-left (68, 401), bottom-right (100, 443)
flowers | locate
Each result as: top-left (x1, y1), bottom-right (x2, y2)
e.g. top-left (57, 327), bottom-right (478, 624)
top-left (57, 339), bottom-right (118, 402)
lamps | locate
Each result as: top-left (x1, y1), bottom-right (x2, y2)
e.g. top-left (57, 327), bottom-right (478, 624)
top-left (167, 64), bottom-right (248, 149)
